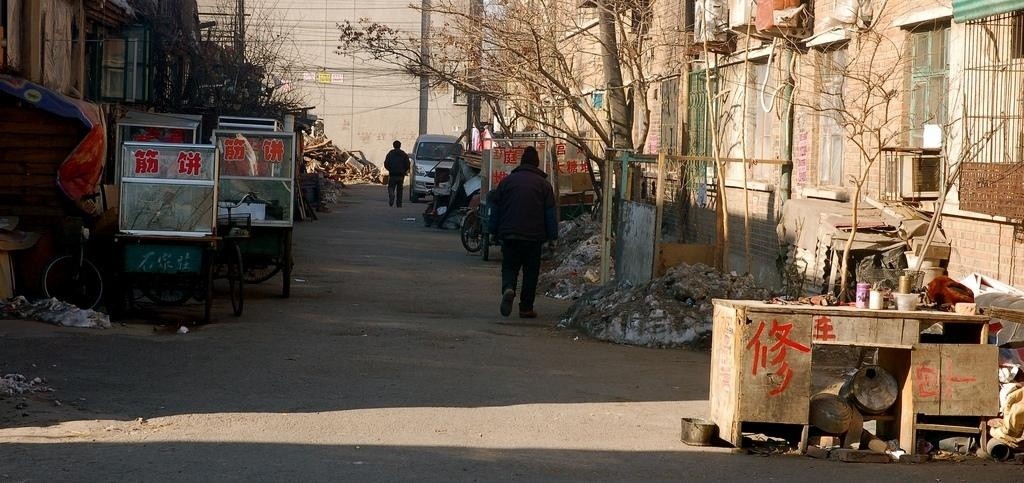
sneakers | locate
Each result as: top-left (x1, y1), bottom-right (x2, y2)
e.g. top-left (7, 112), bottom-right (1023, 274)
top-left (518, 308), bottom-right (537, 318)
top-left (500, 287), bottom-right (514, 317)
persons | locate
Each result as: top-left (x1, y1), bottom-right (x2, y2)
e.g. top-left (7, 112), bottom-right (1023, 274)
top-left (488, 145), bottom-right (560, 319)
top-left (384, 140), bottom-right (410, 208)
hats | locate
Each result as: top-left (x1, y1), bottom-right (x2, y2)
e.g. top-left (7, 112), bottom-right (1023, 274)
top-left (519, 144), bottom-right (539, 167)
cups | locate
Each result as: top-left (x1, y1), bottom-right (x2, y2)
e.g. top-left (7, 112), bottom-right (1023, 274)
top-left (856, 283), bottom-right (890, 310)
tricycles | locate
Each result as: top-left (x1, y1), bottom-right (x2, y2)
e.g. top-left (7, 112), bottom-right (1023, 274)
top-left (39, 111), bottom-right (299, 326)
top-left (461, 145), bottom-right (596, 256)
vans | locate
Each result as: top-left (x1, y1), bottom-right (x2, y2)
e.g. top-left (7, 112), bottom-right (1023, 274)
top-left (409, 133), bottom-right (464, 201)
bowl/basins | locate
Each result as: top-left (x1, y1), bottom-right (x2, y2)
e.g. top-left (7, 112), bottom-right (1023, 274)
top-left (892, 291), bottom-right (920, 310)
